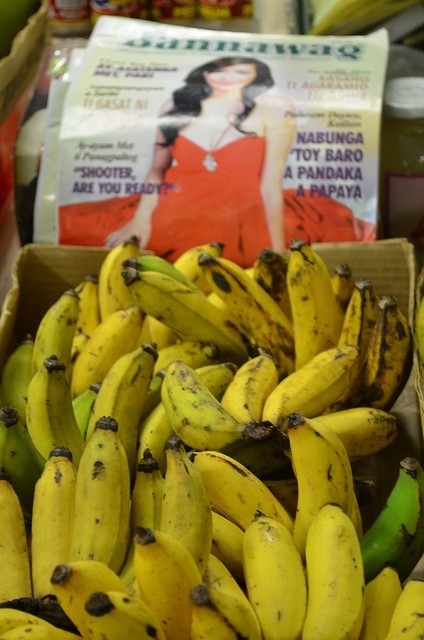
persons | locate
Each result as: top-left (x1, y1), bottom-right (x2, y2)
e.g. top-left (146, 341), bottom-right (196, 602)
top-left (103, 53), bottom-right (295, 269)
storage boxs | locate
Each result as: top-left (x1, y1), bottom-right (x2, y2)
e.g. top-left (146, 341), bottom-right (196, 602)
top-left (0, 238), bottom-right (424, 640)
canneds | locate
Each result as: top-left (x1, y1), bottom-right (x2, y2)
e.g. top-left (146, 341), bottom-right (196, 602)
top-left (200, 1), bottom-right (253, 24)
top-left (87, 1), bottom-right (143, 29)
top-left (150, 2), bottom-right (196, 24)
top-left (46, 0), bottom-right (93, 38)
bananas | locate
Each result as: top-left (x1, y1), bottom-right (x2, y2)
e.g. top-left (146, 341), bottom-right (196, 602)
top-left (1, 237), bottom-right (423, 640)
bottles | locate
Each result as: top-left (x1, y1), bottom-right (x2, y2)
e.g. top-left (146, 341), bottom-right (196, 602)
top-left (379, 74), bottom-right (424, 240)
